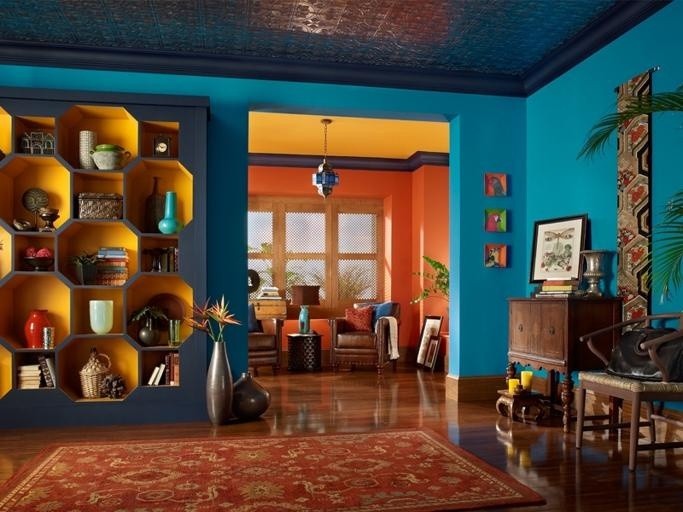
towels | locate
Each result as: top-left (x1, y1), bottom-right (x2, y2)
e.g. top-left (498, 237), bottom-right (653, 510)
top-left (374, 316), bottom-right (400, 361)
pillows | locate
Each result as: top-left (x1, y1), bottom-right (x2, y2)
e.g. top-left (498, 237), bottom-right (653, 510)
top-left (248, 303), bottom-right (259, 332)
top-left (346, 304), bottom-right (374, 332)
top-left (353, 302), bottom-right (392, 329)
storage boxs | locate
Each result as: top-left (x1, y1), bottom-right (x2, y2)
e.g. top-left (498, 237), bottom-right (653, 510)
top-left (252, 300), bottom-right (287, 320)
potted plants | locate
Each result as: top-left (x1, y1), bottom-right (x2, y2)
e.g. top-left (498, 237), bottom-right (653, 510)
top-left (410, 255), bottom-right (449, 355)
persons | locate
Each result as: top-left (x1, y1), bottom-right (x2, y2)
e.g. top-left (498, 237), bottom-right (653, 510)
top-left (540, 251), bottom-right (562, 272)
top-left (558, 244), bottom-right (572, 271)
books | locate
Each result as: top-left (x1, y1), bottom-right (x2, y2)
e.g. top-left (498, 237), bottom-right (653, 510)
top-left (146, 246), bottom-right (179, 273)
top-left (533, 277), bottom-right (577, 298)
top-left (16, 356), bottom-right (56, 389)
top-left (148, 352), bottom-right (180, 387)
top-left (98, 247), bottom-right (129, 286)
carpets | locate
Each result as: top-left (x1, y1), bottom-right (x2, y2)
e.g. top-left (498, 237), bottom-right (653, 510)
top-left (0, 426), bottom-right (546, 512)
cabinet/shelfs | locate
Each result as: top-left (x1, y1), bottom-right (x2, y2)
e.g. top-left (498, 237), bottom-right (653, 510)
top-left (1, 101), bottom-right (207, 429)
top-left (506, 297), bottom-right (623, 433)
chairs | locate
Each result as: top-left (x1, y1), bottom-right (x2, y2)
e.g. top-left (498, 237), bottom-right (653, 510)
top-left (248, 318), bottom-right (282, 376)
top-left (329, 303), bottom-right (401, 375)
top-left (576, 313), bottom-right (683, 470)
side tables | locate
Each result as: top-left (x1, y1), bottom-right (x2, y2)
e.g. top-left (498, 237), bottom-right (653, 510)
top-left (286, 333), bottom-right (324, 371)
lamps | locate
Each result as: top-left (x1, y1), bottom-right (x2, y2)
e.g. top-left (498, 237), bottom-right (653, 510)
top-left (312, 119), bottom-right (339, 200)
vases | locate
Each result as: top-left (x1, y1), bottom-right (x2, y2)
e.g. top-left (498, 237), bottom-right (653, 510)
top-left (207, 342), bottom-right (234, 425)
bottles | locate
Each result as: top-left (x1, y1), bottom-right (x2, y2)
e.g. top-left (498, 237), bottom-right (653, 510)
top-left (145, 177), bottom-right (164, 233)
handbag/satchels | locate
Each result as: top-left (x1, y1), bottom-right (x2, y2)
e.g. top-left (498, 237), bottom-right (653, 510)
top-left (608, 328), bottom-right (682, 382)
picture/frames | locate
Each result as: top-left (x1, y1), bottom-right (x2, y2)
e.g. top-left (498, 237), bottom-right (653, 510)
top-left (423, 335), bottom-right (442, 372)
top-left (528, 213), bottom-right (588, 283)
top-left (414, 315), bottom-right (443, 366)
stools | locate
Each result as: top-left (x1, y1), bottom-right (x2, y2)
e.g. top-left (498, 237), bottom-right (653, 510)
top-left (496, 390), bottom-right (546, 421)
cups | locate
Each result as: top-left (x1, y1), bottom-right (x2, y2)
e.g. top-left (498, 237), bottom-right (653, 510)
top-left (43, 327), bottom-right (54, 349)
top-left (521, 371), bottom-right (533, 390)
top-left (508, 379), bottom-right (519, 394)
top-left (89, 300), bottom-right (114, 334)
top-left (79, 130), bottom-right (97, 170)
top-left (170, 320), bottom-right (180, 346)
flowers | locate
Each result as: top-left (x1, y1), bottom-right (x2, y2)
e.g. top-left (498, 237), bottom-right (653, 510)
top-left (181, 293), bottom-right (243, 341)
